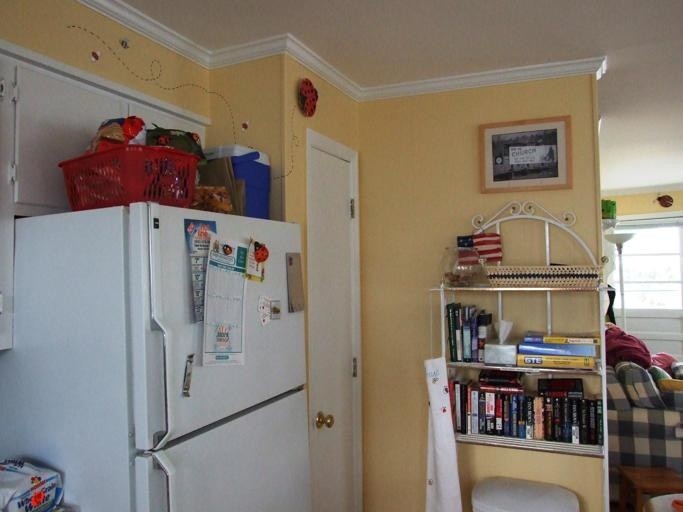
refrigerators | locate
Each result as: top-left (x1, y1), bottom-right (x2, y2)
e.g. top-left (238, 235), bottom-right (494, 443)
top-left (0, 201), bottom-right (306, 512)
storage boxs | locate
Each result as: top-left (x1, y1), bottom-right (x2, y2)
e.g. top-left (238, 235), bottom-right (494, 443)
top-left (202, 145), bottom-right (270, 219)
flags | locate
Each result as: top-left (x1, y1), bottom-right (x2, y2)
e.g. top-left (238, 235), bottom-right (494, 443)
top-left (455, 229), bottom-right (503, 265)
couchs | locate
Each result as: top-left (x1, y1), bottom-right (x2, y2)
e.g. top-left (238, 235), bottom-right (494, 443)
top-left (608, 360), bottom-right (683, 504)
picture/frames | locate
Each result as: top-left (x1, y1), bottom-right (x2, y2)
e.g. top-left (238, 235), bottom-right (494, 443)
top-left (479, 115), bottom-right (573, 194)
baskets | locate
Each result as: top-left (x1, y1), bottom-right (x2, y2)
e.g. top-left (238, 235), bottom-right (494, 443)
top-left (57, 143), bottom-right (202, 212)
top-left (479, 256), bottom-right (611, 288)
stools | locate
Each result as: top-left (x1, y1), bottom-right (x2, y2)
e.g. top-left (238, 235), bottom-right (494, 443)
top-left (618, 464), bottom-right (683, 512)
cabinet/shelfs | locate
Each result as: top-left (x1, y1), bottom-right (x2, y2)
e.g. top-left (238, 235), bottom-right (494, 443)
top-left (0, 52), bottom-right (14, 350)
top-left (12, 64), bottom-right (206, 217)
top-left (427, 200), bottom-right (611, 512)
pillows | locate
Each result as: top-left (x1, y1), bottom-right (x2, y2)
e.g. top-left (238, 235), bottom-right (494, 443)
top-left (616, 358), bottom-right (683, 409)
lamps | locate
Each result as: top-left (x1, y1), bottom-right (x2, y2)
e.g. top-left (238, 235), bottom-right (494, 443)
top-left (606, 233), bottom-right (635, 331)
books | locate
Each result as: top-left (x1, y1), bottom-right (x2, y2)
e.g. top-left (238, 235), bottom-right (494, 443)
top-left (443, 301), bottom-right (605, 446)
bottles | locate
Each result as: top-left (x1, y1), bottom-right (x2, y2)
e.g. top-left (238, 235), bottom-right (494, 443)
top-left (442, 246), bottom-right (482, 288)
